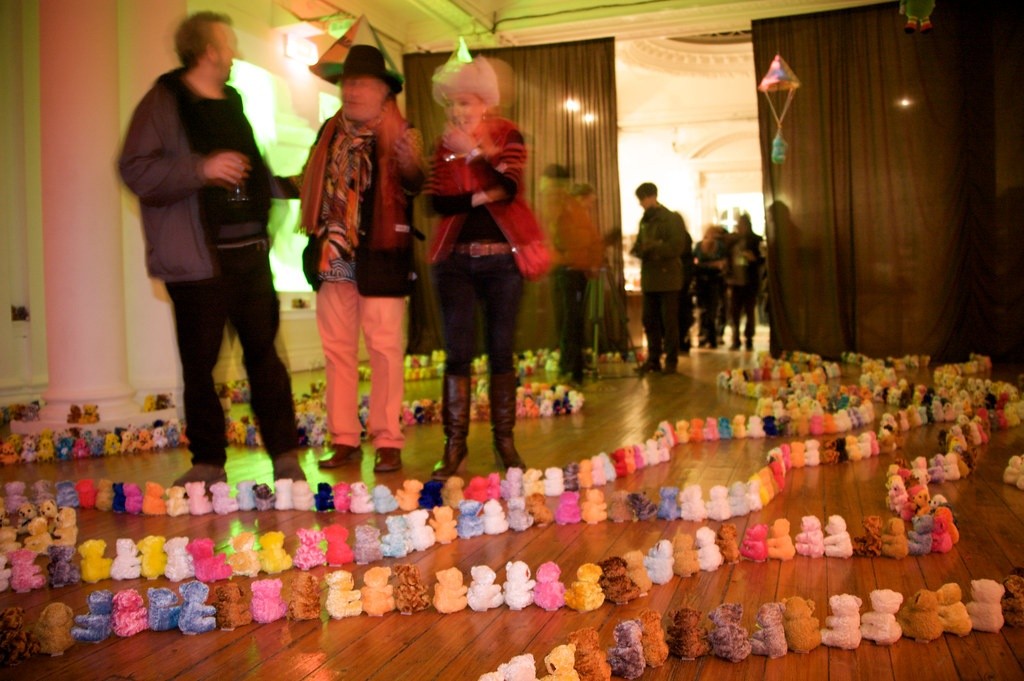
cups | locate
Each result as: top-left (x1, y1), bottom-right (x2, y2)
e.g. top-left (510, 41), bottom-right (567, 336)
top-left (228, 180), bottom-right (249, 202)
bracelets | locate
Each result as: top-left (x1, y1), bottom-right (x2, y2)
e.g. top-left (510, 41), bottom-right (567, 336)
top-left (465, 148), bottom-right (483, 164)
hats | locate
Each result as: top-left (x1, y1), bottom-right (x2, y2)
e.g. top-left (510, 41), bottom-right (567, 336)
top-left (434, 51), bottom-right (501, 107)
top-left (325, 46), bottom-right (402, 92)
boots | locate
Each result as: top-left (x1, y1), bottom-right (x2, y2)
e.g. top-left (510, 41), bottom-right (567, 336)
top-left (490, 371), bottom-right (526, 475)
top-left (431, 375), bottom-right (472, 480)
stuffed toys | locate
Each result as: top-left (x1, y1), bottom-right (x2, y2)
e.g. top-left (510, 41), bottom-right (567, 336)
top-left (0, 349), bottom-right (1024, 681)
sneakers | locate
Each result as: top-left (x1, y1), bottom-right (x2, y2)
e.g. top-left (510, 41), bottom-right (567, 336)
top-left (272, 451), bottom-right (305, 481)
top-left (173, 462), bottom-right (227, 490)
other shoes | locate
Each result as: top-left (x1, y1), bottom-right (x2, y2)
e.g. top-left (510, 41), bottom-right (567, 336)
top-left (729, 339), bottom-right (741, 350)
top-left (745, 339), bottom-right (754, 351)
top-left (637, 362), bottom-right (659, 375)
top-left (661, 366), bottom-right (674, 376)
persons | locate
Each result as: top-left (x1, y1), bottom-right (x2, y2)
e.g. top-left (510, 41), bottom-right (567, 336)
top-left (119, 11), bottom-right (305, 484)
top-left (630, 182), bottom-right (686, 375)
top-left (296, 44), bottom-right (428, 471)
top-left (430, 56), bottom-right (550, 480)
top-left (672, 210), bottom-right (767, 352)
top-left (552, 182), bottom-right (607, 391)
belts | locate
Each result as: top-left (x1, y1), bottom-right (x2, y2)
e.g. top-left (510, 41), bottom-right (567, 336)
top-left (452, 240), bottom-right (513, 258)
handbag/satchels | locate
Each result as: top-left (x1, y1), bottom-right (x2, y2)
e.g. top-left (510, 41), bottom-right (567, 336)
top-left (354, 249), bottom-right (417, 297)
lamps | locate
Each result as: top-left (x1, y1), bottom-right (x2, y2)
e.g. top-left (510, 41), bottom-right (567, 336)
top-left (298, 10), bottom-right (358, 38)
top-left (431, 29), bottom-right (489, 86)
top-left (758, 19), bottom-right (802, 165)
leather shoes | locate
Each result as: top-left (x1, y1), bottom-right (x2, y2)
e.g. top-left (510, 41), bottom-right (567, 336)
top-left (318, 446), bottom-right (362, 468)
top-left (372, 447), bottom-right (402, 470)
